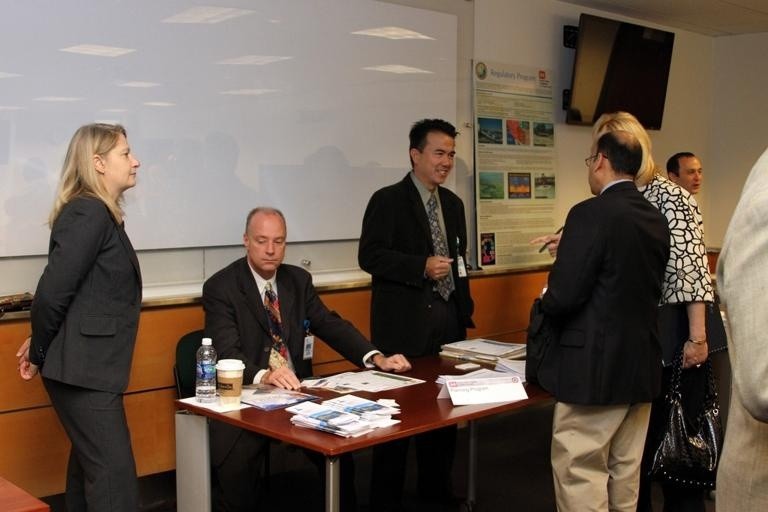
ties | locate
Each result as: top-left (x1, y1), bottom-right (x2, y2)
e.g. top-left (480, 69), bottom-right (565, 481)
top-left (262, 283), bottom-right (289, 369)
top-left (427, 191), bottom-right (453, 300)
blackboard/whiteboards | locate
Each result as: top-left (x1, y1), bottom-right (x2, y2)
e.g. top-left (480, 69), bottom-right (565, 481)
top-left (0, 0), bottom-right (459, 260)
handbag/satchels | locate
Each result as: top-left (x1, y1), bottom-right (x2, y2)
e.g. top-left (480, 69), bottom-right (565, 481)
top-left (646, 399), bottom-right (723, 493)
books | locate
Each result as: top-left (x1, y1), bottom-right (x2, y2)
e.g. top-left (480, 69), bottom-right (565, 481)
top-left (441, 339), bottom-right (526, 362)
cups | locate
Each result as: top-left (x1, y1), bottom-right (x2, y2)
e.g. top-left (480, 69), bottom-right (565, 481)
top-left (216, 358), bottom-right (246, 405)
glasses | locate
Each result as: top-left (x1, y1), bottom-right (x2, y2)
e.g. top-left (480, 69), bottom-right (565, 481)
top-left (583, 155), bottom-right (608, 168)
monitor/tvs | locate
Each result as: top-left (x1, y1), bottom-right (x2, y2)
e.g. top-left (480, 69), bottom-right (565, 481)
top-left (561, 12), bottom-right (675, 131)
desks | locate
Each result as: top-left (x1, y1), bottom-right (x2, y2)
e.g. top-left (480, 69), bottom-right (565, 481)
top-left (173, 344), bottom-right (552, 511)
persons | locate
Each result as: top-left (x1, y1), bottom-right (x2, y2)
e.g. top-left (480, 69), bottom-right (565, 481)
top-left (200, 204), bottom-right (414, 395)
top-left (357, 115), bottom-right (477, 358)
top-left (663, 150), bottom-right (708, 199)
top-left (540, 128), bottom-right (673, 512)
top-left (526, 108), bottom-right (724, 512)
top-left (712, 143), bottom-right (768, 512)
top-left (15, 120), bottom-right (143, 511)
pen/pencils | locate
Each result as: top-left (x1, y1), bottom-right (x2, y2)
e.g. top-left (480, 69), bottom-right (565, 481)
top-left (540, 226), bottom-right (565, 252)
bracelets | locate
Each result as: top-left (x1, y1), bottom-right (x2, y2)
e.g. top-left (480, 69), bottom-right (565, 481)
top-left (687, 337), bottom-right (711, 348)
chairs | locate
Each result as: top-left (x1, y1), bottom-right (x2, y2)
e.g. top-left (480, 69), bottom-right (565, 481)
top-left (175, 328), bottom-right (272, 485)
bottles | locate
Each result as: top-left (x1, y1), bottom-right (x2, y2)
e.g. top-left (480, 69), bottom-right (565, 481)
top-left (195, 337), bottom-right (217, 402)
